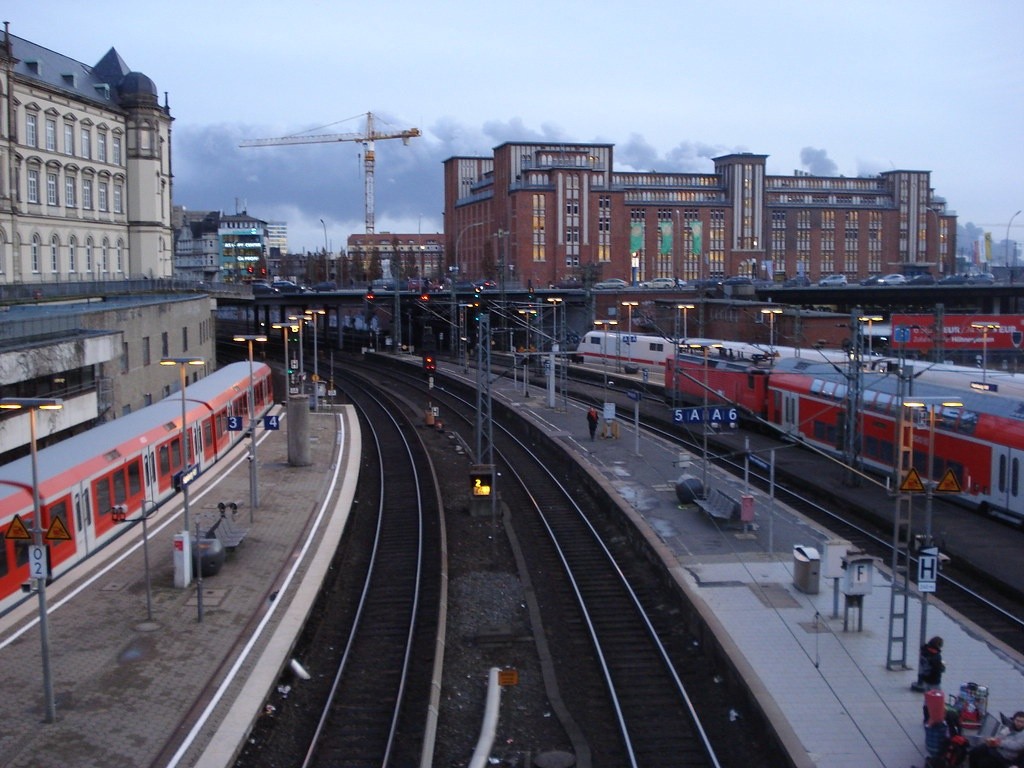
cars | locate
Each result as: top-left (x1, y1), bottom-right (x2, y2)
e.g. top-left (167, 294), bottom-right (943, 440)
top-left (384, 279), bottom-right (498, 291)
top-left (549, 272), bottom-right (995, 290)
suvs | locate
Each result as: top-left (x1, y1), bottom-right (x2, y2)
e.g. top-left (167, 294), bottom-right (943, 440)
top-left (242, 279), bottom-right (337, 294)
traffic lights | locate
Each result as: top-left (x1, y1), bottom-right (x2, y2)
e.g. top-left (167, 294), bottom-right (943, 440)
top-left (423, 352), bottom-right (436, 374)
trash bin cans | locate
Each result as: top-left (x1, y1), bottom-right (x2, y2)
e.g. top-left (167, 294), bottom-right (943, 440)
top-left (793, 545), bottom-right (820, 594)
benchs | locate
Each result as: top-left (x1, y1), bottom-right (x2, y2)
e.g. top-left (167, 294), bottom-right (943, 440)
top-left (194, 515), bottom-right (251, 552)
top-left (693, 488), bottom-right (759, 525)
top-left (962, 713), bottom-right (1014, 738)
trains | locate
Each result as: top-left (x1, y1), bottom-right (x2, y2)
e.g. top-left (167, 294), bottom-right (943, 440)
top-left (574, 331), bottom-right (1024, 527)
top-left (0, 361), bottom-right (274, 610)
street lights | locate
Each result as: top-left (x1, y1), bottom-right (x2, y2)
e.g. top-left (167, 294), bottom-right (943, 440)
top-left (0, 397), bottom-right (62, 724)
top-left (762, 309), bottom-right (783, 356)
top-left (455, 222), bottom-right (484, 278)
top-left (1006, 211), bottom-right (1021, 272)
top-left (320, 219), bottom-right (327, 283)
top-left (519, 309), bottom-right (536, 399)
top-left (972, 322), bottom-right (1001, 385)
top-left (903, 394), bottom-right (964, 692)
top-left (678, 305), bottom-right (694, 345)
top-left (860, 316), bottom-right (883, 371)
top-left (622, 301), bottom-right (638, 363)
top-left (548, 298), bottom-right (562, 345)
top-left (160, 357), bottom-right (204, 583)
top-left (593, 319), bottom-right (617, 439)
top-left (233, 334), bottom-right (267, 510)
top-left (272, 310), bottom-right (325, 464)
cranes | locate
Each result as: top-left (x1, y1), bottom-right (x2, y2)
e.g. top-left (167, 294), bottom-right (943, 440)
top-left (240, 111), bottom-right (423, 244)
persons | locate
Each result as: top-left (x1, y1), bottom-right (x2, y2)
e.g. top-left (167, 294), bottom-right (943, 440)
top-left (672, 273), bottom-right (681, 290)
top-left (587, 406), bottom-right (599, 441)
top-left (969, 711), bottom-right (1024, 768)
top-left (423, 277), bottom-right (430, 293)
top-left (919, 636), bottom-right (946, 723)
